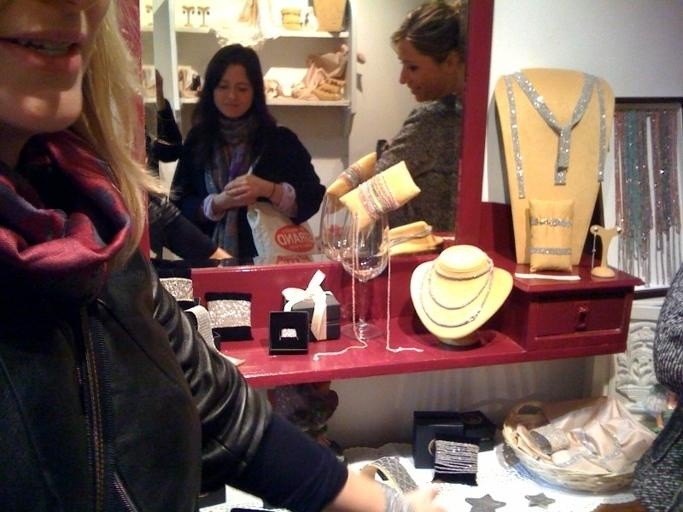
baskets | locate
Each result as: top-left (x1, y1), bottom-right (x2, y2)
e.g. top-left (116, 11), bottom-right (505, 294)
top-left (502, 398), bottom-right (660, 495)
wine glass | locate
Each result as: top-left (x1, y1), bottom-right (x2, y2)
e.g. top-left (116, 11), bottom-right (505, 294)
top-left (338, 209), bottom-right (391, 341)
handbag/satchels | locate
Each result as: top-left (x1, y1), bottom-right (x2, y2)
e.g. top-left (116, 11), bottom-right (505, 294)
top-left (242, 200), bottom-right (318, 261)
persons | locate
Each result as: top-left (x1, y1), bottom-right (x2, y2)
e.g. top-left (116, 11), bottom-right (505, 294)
top-left (147, 184), bottom-right (233, 259)
top-left (1, 0), bottom-right (447, 512)
top-left (146, 67), bottom-right (184, 179)
top-left (371, 0), bottom-right (468, 231)
top-left (627, 263), bottom-right (683, 511)
top-left (169, 41), bottom-right (328, 258)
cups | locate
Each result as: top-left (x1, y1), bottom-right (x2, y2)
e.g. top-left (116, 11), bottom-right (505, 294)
top-left (319, 194), bottom-right (357, 263)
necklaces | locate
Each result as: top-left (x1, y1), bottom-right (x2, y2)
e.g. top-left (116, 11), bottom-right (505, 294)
top-left (421, 256), bottom-right (494, 328)
top-left (513, 68), bottom-right (598, 187)
top-left (387, 226), bottom-right (435, 246)
top-left (428, 261), bottom-right (493, 310)
top-left (435, 261), bottom-right (491, 280)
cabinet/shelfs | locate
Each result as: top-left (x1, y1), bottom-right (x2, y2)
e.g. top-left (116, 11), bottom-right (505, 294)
top-left (134, 23), bottom-right (350, 116)
top-left (488, 291), bottom-right (630, 354)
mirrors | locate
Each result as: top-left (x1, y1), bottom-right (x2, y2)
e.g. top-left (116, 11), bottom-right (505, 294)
top-left (107, 0), bottom-right (499, 280)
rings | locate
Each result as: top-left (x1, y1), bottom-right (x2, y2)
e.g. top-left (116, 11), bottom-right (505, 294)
top-left (277, 328), bottom-right (301, 343)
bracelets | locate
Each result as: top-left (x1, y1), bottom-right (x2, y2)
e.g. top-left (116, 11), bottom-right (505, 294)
top-left (338, 162), bottom-right (367, 192)
top-left (530, 423), bottom-right (569, 454)
top-left (160, 276), bottom-right (195, 304)
top-left (358, 172), bottom-right (399, 219)
top-left (433, 439), bottom-right (479, 477)
top-left (205, 299), bottom-right (252, 330)
top-left (529, 216), bottom-right (574, 229)
top-left (384, 483), bottom-right (408, 512)
top-left (267, 181), bottom-right (279, 200)
top-left (530, 247), bottom-right (572, 255)
